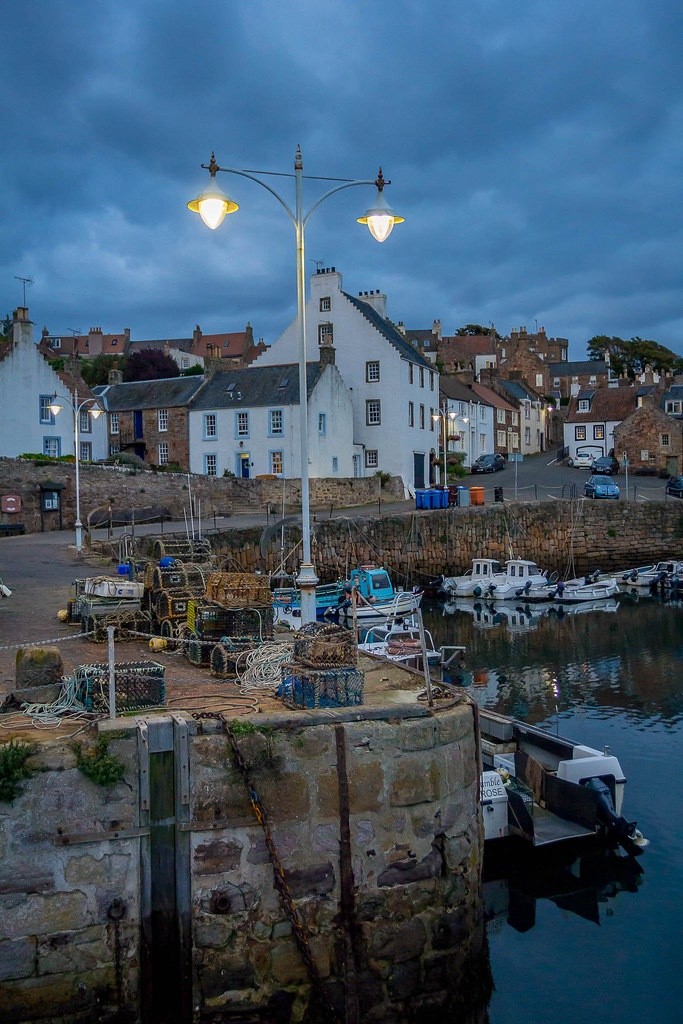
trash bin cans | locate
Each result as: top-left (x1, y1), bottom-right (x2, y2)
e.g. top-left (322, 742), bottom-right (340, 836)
top-left (469, 486), bottom-right (485, 506)
top-left (415, 490), bottom-right (443, 510)
top-left (494, 486), bottom-right (503, 502)
top-left (435, 484), bottom-right (457, 506)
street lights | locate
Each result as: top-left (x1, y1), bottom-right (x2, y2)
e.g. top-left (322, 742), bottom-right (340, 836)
top-left (186, 144), bottom-right (407, 625)
top-left (431, 406), bottom-right (469, 489)
top-left (40, 389), bottom-right (104, 552)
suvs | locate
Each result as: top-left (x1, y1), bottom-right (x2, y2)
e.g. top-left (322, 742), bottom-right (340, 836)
top-left (574, 453), bottom-right (596, 469)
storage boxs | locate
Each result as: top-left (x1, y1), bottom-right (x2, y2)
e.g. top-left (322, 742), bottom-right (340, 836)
top-left (481, 732), bottom-right (516, 778)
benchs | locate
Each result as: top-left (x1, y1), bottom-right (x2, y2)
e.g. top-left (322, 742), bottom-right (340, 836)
top-left (0, 524), bottom-right (24, 537)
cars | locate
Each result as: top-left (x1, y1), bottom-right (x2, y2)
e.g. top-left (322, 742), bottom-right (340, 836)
top-left (590, 457), bottom-right (619, 476)
top-left (584, 475), bottom-right (620, 500)
top-left (666, 475), bottom-right (683, 499)
top-left (471, 453), bottom-right (504, 474)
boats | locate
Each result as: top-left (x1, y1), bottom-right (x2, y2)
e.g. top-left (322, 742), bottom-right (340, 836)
top-left (329, 564), bottom-right (424, 619)
top-left (270, 584), bottom-right (348, 634)
top-left (435, 595), bottom-right (620, 636)
top-left (356, 592), bottom-right (441, 669)
top-left (589, 558), bottom-right (683, 597)
top-left (479, 706), bottom-right (649, 860)
top-left (438, 559), bottom-right (616, 600)
